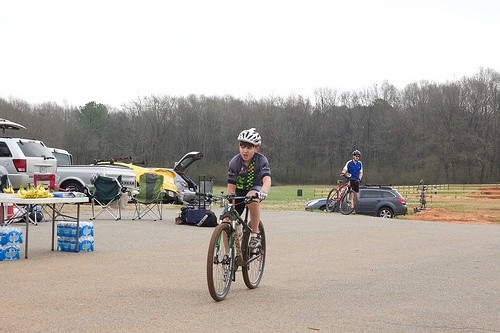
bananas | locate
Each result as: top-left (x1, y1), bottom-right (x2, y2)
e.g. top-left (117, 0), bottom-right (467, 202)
top-left (3, 185), bottom-right (14, 194)
top-left (17, 183), bottom-right (53, 198)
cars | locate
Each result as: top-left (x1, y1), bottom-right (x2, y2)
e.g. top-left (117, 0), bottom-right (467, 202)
top-left (49, 147), bottom-right (204, 202)
top-left (47, 146), bottom-right (73, 167)
top-left (0, 138), bottom-right (58, 189)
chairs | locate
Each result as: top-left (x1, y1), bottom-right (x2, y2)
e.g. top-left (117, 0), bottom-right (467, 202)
top-left (82, 173), bottom-right (127, 221)
top-left (6, 174), bottom-right (67, 225)
top-left (126, 173), bottom-right (165, 221)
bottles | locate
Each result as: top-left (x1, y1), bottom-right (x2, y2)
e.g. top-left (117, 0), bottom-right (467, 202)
top-left (0, 244), bottom-right (20, 261)
top-left (56, 222), bottom-right (95, 237)
top-left (0, 226), bottom-right (23, 244)
top-left (57, 237), bottom-right (95, 251)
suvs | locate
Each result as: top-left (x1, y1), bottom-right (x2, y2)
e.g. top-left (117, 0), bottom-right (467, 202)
top-left (304, 184), bottom-right (407, 218)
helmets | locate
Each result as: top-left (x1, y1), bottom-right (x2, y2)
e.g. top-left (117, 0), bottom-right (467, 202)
top-left (352, 150), bottom-right (361, 154)
top-left (237, 127), bottom-right (261, 145)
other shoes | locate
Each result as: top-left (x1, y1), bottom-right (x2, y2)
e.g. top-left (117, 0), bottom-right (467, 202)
top-left (249, 231), bottom-right (261, 247)
top-left (222, 255), bottom-right (231, 264)
top-left (350, 207), bottom-right (355, 214)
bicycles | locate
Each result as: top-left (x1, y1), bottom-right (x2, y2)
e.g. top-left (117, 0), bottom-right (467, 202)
top-left (326, 174), bottom-right (360, 215)
top-left (195, 192), bottom-right (266, 302)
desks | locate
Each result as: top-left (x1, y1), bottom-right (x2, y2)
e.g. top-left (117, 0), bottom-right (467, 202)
top-left (1, 192), bottom-right (89, 259)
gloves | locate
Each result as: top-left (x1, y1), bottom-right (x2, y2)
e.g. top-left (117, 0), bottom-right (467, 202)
top-left (257, 191), bottom-right (268, 200)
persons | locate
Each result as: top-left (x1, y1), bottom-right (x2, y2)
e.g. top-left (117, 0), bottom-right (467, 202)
top-left (216, 127), bottom-right (271, 281)
top-left (342, 149), bottom-right (363, 214)
